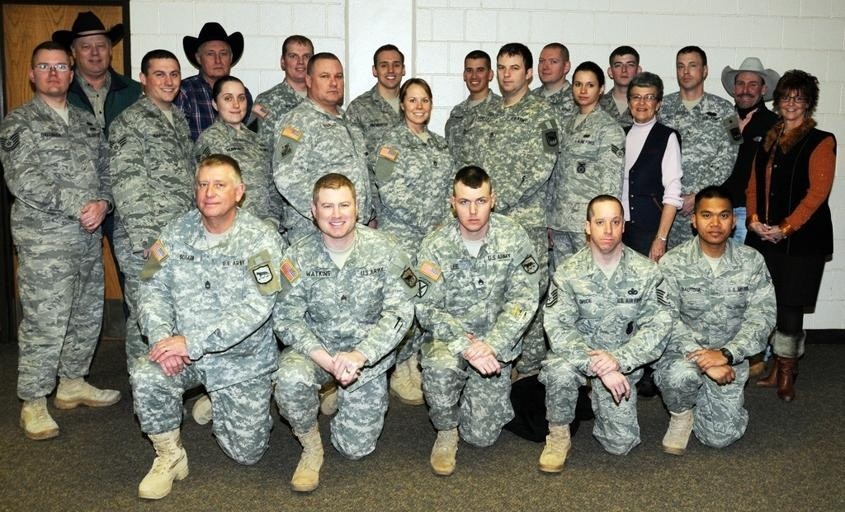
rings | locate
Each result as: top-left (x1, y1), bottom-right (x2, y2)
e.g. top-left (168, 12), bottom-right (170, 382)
top-left (774, 238), bottom-right (778, 242)
top-left (346, 368), bottom-right (349, 374)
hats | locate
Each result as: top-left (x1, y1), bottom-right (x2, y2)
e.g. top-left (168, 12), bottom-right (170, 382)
top-left (181, 22), bottom-right (244, 69)
top-left (52, 10), bottom-right (125, 57)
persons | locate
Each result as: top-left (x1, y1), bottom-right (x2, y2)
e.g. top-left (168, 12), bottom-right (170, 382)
top-left (343, 45), bottom-right (405, 230)
top-left (130, 153), bottom-right (288, 499)
top-left (415, 164), bottom-right (539, 475)
top-left (532, 43), bottom-right (580, 132)
top-left (271, 172), bottom-right (416, 492)
top-left (174, 22), bottom-right (254, 144)
top-left (539, 194), bottom-right (672, 474)
top-left (743, 69), bottom-right (837, 401)
top-left (1, 42), bottom-right (124, 442)
top-left (271, 53), bottom-right (377, 417)
top-left (621, 72), bottom-right (683, 263)
top-left (52, 11), bottom-right (143, 321)
top-left (445, 43), bottom-right (562, 385)
top-left (192, 77), bottom-right (283, 232)
top-left (722, 58), bottom-right (782, 246)
top-left (371, 77), bottom-right (458, 406)
top-left (548, 60), bottom-right (624, 269)
top-left (248, 36), bottom-right (315, 146)
top-left (445, 50), bottom-right (502, 156)
top-left (650, 185), bottom-right (776, 456)
top-left (109, 52), bottom-right (199, 316)
top-left (656, 46), bottom-right (740, 253)
top-left (600, 46), bottom-right (641, 130)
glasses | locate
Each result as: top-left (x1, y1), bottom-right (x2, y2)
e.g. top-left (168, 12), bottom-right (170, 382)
top-left (782, 95), bottom-right (805, 104)
top-left (627, 94), bottom-right (659, 103)
top-left (30, 62), bottom-right (71, 75)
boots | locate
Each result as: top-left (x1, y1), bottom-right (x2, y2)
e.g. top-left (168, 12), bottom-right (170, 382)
top-left (539, 423), bottom-right (572, 473)
top-left (322, 387), bottom-right (341, 417)
top-left (138, 428), bottom-right (189, 500)
top-left (721, 56), bottom-right (781, 102)
top-left (664, 405), bottom-right (696, 457)
top-left (55, 377), bottom-right (122, 411)
top-left (18, 392), bottom-right (61, 442)
top-left (755, 355), bottom-right (778, 387)
top-left (391, 349), bottom-right (428, 409)
top-left (429, 429), bottom-right (460, 479)
top-left (292, 423), bottom-right (325, 494)
top-left (777, 357), bottom-right (798, 404)
top-left (193, 397), bottom-right (212, 428)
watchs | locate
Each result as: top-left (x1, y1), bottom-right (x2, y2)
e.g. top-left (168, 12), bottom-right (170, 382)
top-left (720, 348), bottom-right (733, 366)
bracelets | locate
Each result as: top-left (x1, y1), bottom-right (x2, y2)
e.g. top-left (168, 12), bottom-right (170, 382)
top-left (656, 235), bottom-right (667, 242)
